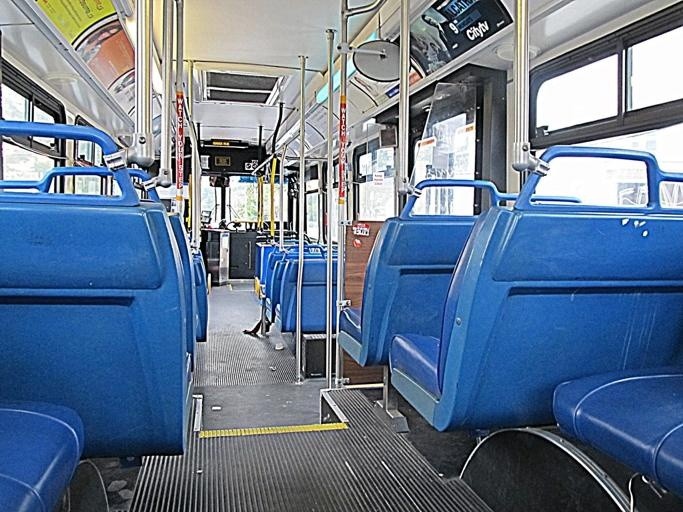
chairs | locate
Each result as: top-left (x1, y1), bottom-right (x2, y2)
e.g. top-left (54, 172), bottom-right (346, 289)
top-left (388, 145), bottom-right (683, 481)
top-left (0, 119), bottom-right (194, 467)
top-left (192, 249), bottom-right (208, 342)
top-left (253, 232), bottom-right (338, 334)
top-left (0, 397), bottom-right (111, 512)
top-left (337, 179), bottom-right (582, 433)
top-left (0, 167), bottom-right (199, 372)
top-left (553, 365), bottom-right (683, 512)
